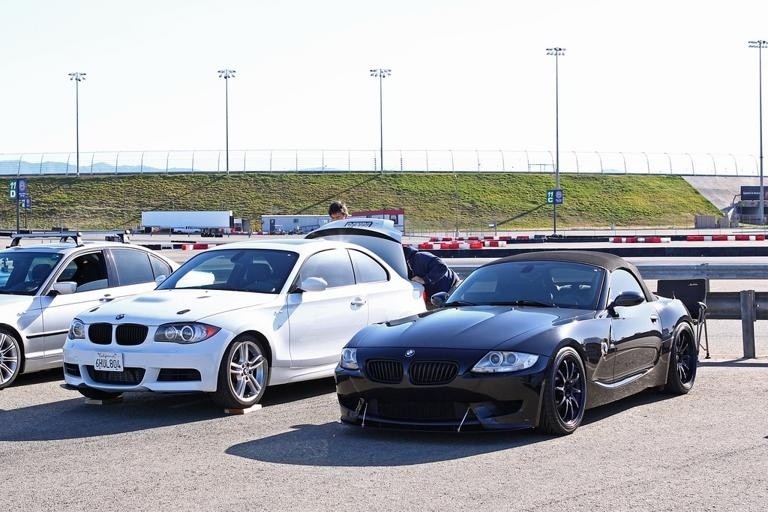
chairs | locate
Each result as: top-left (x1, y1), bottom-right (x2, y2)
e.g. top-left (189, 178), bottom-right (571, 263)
top-left (233, 257), bottom-right (280, 295)
top-left (7, 260), bottom-right (56, 294)
top-left (643, 276), bottom-right (713, 364)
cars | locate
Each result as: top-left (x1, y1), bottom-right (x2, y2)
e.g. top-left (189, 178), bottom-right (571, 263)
top-left (0, 231), bottom-right (216, 383)
top-left (64, 218), bottom-right (432, 406)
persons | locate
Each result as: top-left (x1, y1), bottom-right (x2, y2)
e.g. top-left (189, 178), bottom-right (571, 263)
top-left (402, 246), bottom-right (465, 310)
top-left (328, 202), bottom-right (349, 222)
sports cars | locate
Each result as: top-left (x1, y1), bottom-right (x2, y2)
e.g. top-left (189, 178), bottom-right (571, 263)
top-left (337, 250), bottom-right (703, 438)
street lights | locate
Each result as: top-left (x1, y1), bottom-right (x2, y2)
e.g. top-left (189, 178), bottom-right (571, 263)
top-left (66, 65), bottom-right (88, 177)
top-left (544, 46), bottom-right (566, 233)
top-left (218, 65), bottom-right (237, 173)
top-left (369, 66), bottom-right (396, 173)
top-left (747, 38), bottom-right (767, 226)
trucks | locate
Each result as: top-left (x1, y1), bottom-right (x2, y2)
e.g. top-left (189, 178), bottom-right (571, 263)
top-left (731, 185), bottom-right (767, 227)
